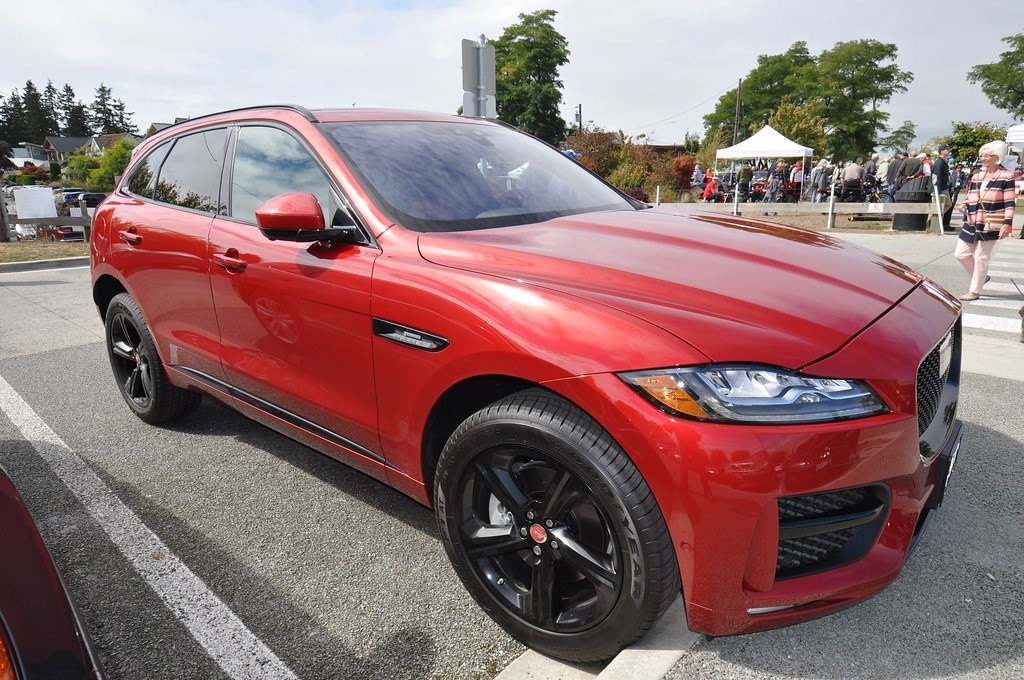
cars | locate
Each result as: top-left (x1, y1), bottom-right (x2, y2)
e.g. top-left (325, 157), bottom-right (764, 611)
top-left (1, 185), bottom-right (107, 242)
top-left (90, 105), bottom-right (970, 664)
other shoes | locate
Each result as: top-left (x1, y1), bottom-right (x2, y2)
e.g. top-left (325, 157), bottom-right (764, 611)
top-left (984, 275), bottom-right (990, 283)
top-left (959, 292), bottom-right (980, 301)
top-left (944, 225), bottom-right (955, 231)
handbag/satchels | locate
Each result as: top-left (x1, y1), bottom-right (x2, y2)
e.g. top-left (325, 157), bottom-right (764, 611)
top-left (983, 212), bottom-right (1006, 232)
top-left (813, 182), bottom-right (818, 188)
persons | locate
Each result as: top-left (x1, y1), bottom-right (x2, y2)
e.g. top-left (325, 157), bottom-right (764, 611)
top-left (1014, 161), bottom-right (1024, 195)
top-left (689, 148), bottom-right (932, 216)
top-left (564, 150), bottom-right (582, 160)
top-left (955, 140), bottom-right (1015, 300)
top-left (927, 145), bottom-right (969, 231)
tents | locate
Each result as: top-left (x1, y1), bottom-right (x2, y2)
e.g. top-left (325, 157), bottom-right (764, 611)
top-left (1006, 123), bottom-right (1024, 155)
top-left (713, 125), bottom-right (814, 202)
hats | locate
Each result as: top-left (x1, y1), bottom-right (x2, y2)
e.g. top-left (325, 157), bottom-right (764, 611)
top-left (938, 145), bottom-right (953, 151)
top-left (712, 177), bottom-right (720, 180)
